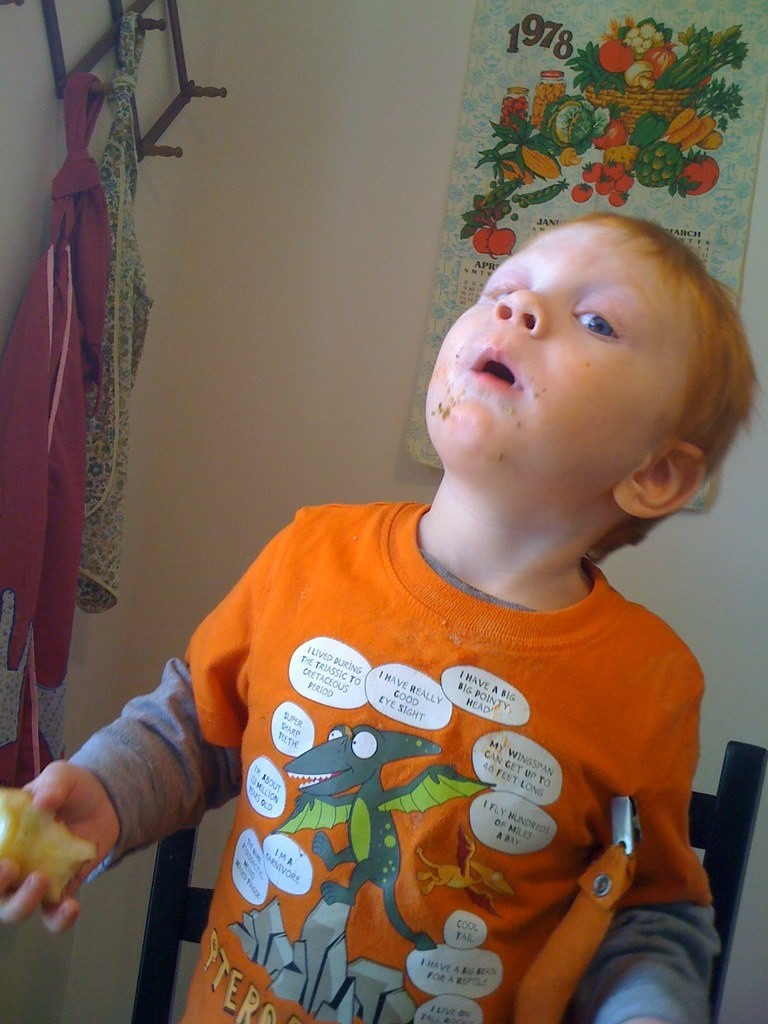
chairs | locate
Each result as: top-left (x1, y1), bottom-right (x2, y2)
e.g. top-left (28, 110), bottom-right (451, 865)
top-left (130, 741), bottom-right (768, 1024)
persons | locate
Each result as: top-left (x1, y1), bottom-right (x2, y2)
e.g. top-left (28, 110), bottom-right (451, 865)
top-left (1, 208), bottom-right (764, 1024)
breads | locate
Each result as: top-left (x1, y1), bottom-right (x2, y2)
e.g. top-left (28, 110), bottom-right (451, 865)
top-left (0, 786), bottom-right (101, 904)
